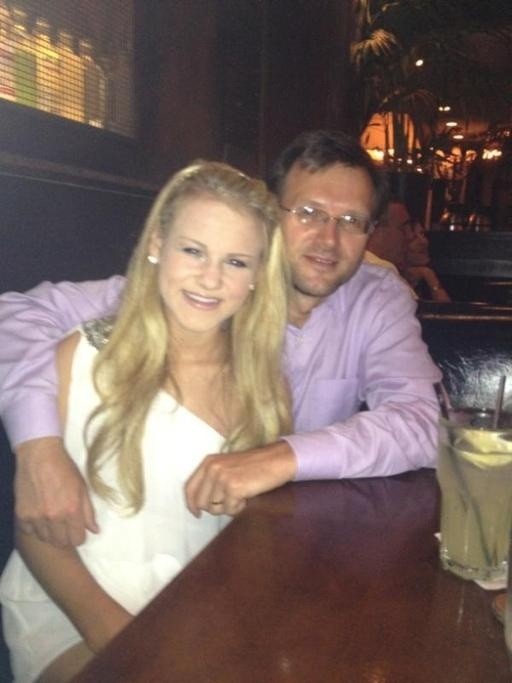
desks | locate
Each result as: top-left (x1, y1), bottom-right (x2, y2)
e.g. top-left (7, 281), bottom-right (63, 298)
top-left (63, 466), bottom-right (509, 683)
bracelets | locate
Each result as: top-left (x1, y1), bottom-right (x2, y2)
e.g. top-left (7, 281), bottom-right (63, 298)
top-left (430, 284), bottom-right (443, 294)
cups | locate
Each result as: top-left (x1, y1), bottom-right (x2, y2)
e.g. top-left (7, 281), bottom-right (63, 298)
top-left (434, 406), bottom-right (512, 594)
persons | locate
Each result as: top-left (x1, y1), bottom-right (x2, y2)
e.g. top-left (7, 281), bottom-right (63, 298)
top-left (0, 157), bottom-right (298, 683)
top-left (362, 193), bottom-right (419, 302)
top-left (0, 127), bottom-right (450, 548)
top-left (402, 219), bottom-right (450, 307)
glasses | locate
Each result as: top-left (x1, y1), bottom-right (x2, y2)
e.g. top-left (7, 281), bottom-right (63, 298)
top-left (278, 204), bottom-right (376, 236)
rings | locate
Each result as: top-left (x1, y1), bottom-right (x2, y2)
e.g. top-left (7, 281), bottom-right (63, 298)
top-left (212, 494), bottom-right (226, 508)
